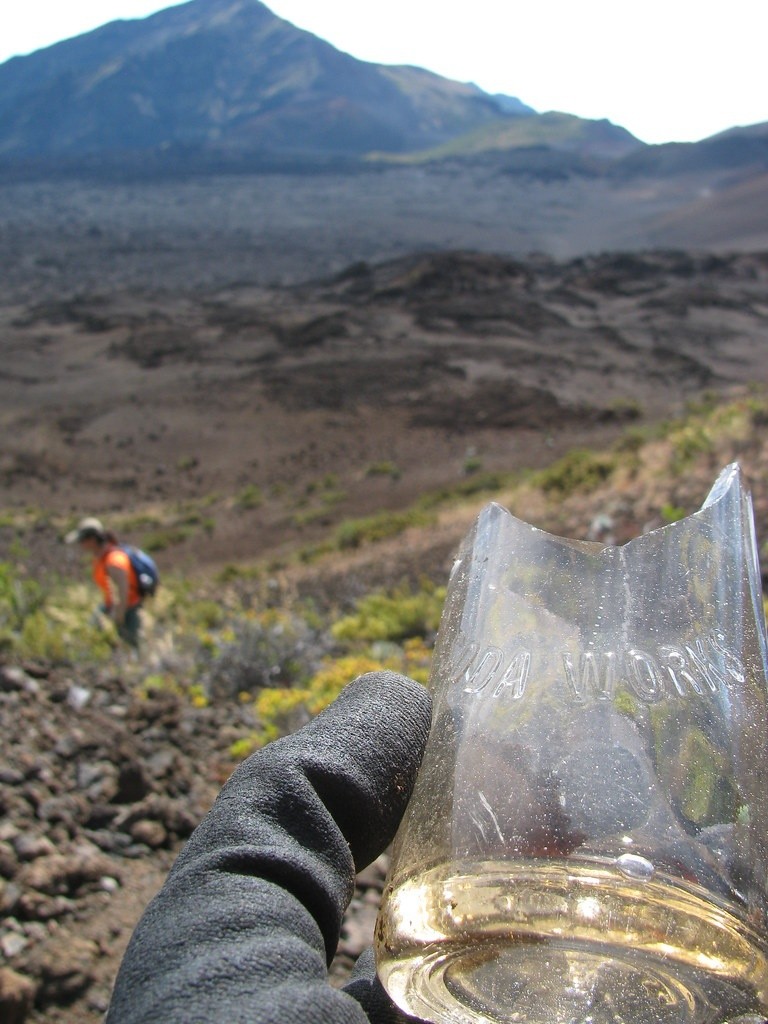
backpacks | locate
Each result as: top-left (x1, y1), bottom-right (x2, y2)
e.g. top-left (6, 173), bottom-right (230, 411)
top-left (122, 545), bottom-right (158, 597)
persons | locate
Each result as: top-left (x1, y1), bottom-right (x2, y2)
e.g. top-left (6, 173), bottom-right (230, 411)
top-left (76, 517), bottom-right (145, 660)
top-left (101, 672), bottom-right (768, 1022)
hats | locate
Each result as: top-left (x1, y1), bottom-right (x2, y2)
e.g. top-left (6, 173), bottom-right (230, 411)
top-left (64, 518), bottom-right (104, 544)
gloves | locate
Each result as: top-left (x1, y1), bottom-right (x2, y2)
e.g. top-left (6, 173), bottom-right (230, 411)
top-left (107, 669), bottom-right (767, 1023)
